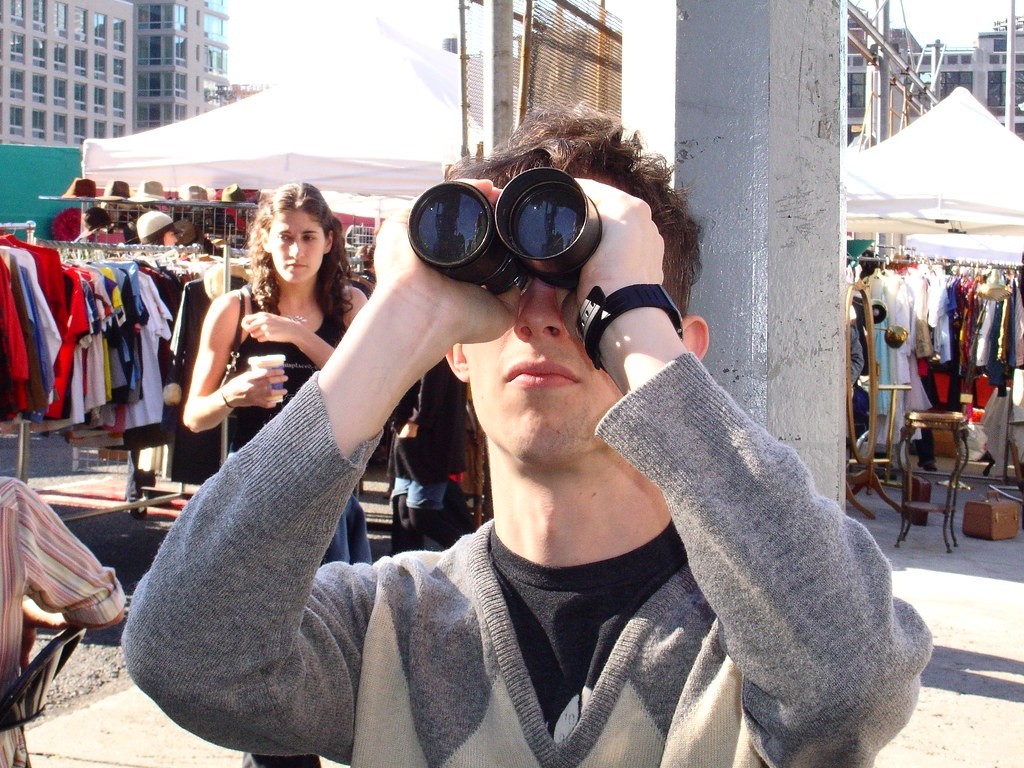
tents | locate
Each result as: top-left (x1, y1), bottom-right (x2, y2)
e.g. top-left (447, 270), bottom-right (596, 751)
top-left (80, 59), bottom-right (495, 205)
top-left (844, 86), bottom-right (1024, 233)
top-left (904, 233), bottom-right (1024, 267)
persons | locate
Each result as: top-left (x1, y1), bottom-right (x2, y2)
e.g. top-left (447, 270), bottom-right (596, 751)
top-left (0, 475), bottom-right (127, 767)
top-left (390, 357), bottom-right (471, 553)
top-left (355, 242), bottom-right (377, 289)
top-left (181, 181), bottom-right (369, 768)
top-left (80, 207), bottom-right (114, 244)
top-left (848, 303), bottom-right (871, 446)
top-left (126, 210), bottom-right (181, 520)
top-left (121, 105), bottom-right (937, 768)
top-left (912, 429), bottom-right (938, 472)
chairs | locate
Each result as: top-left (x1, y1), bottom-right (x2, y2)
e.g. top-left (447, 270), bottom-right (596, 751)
top-left (0, 626), bottom-right (88, 732)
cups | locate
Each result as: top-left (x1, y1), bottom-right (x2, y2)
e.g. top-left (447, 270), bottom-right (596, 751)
top-left (248, 354), bottom-right (287, 403)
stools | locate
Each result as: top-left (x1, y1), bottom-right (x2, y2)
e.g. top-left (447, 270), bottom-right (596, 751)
top-left (896, 410), bottom-right (968, 553)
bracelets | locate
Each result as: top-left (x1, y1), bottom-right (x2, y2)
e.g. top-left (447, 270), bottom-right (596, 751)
top-left (221, 386), bottom-right (235, 408)
top-left (575, 280), bottom-right (684, 373)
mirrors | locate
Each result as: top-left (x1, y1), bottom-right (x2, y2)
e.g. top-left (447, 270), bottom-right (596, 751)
top-left (846, 278), bottom-right (902, 520)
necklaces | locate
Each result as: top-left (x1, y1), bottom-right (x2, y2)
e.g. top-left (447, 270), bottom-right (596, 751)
top-left (276, 294), bottom-right (317, 323)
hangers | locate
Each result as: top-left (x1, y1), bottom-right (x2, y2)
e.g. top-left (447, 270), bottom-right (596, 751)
top-left (40, 240), bottom-right (206, 265)
top-left (862, 244), bottom-right (1019, 284)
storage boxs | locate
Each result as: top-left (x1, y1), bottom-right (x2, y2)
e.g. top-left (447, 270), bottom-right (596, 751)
top-left (962, 491), bottom-right (1019, 541)
